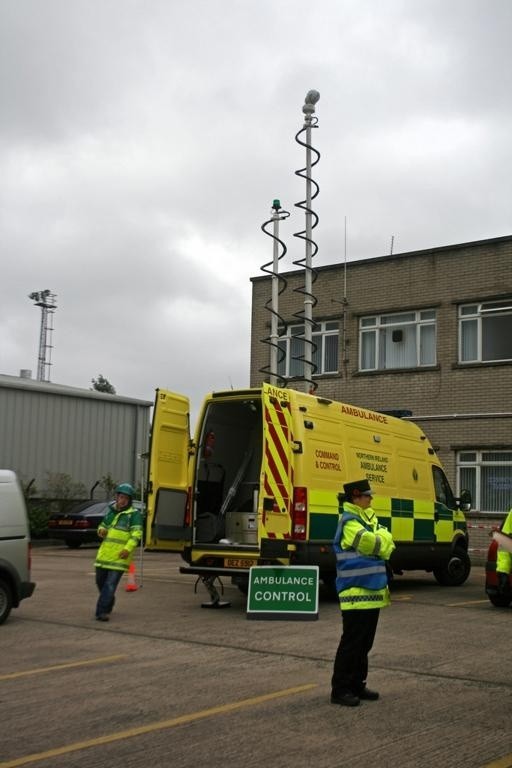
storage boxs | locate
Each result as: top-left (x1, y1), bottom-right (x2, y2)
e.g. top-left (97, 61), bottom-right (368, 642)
top-left (241, 529), bottom-right (257, 544)
top-left (225, 512), bottom-right (257, 544)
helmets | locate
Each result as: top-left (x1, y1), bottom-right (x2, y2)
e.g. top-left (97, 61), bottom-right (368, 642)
top-left (114, 482), bottom-right (135, 496)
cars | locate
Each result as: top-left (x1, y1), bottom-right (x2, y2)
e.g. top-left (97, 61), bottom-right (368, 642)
top-left (49, 498), bottom-right (148, 550)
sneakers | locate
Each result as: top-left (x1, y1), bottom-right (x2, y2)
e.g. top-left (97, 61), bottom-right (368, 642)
top-left (94, 608), bottom-right (113, 621)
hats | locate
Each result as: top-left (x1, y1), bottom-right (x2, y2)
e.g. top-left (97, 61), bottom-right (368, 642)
top-left (343, 479), bottom-right (378, 496)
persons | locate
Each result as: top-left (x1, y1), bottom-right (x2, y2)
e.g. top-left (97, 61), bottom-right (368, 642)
top-left (90, 482), bottom-right (142, 622)
top-left (329, 481), bottom-right (395, 708)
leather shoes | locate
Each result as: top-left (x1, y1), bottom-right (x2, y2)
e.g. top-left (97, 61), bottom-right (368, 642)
top-left (330, 681), bottom-right (380, 706)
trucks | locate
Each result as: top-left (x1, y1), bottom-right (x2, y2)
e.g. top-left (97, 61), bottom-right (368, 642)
top-left (144, 382), bottom-right (474, 610)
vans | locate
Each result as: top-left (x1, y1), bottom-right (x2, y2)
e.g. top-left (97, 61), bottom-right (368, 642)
top-left (484, 508), bottom-right (512, 606)
top-left (1, 469), bottom-right (35, 625)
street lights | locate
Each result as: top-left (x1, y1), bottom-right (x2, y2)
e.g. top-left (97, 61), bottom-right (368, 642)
top-left (24, 288), bottom-right (59, 386)
top-left (292, 88), bottom-right (320, 396)
top-left (260, 198), bottom-right (291, 388)
